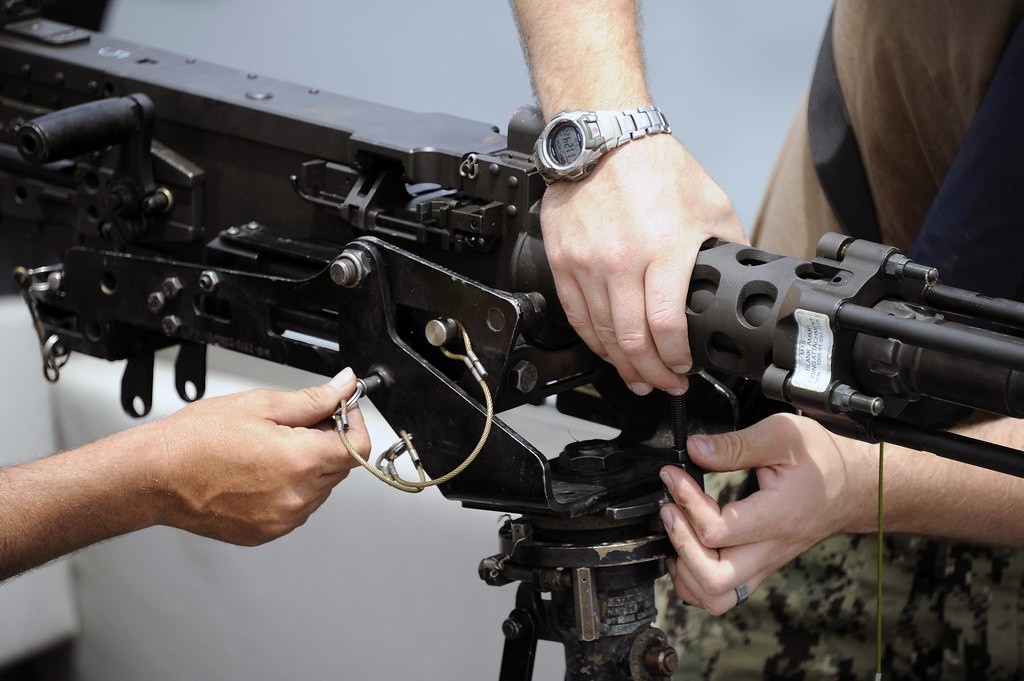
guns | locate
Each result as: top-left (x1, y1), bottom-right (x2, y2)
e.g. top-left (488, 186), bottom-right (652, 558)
top-left (2, 14), bottom-right (1023, 481)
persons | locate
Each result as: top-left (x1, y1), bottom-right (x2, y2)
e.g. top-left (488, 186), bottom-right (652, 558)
top-left (0, 366), bottom-right (371, 584)
top-left (510, 0), bottom-right (1024, 616)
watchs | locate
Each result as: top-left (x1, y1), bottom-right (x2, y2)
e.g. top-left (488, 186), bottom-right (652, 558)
top-left (532, 105), bottom-right (672, 184)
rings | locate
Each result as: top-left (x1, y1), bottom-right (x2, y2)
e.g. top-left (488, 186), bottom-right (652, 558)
top-left (735, 583), bottom-right (749, 605)
